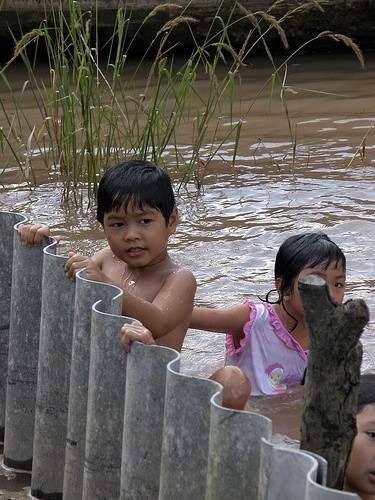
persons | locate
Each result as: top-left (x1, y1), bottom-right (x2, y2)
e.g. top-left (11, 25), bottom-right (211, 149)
top-left (18, 159), bottom-right (196, 353)
top-left (120, 320), bottom-right (375, 500)
top-left (188, 233), bottom-right (346, 412)
